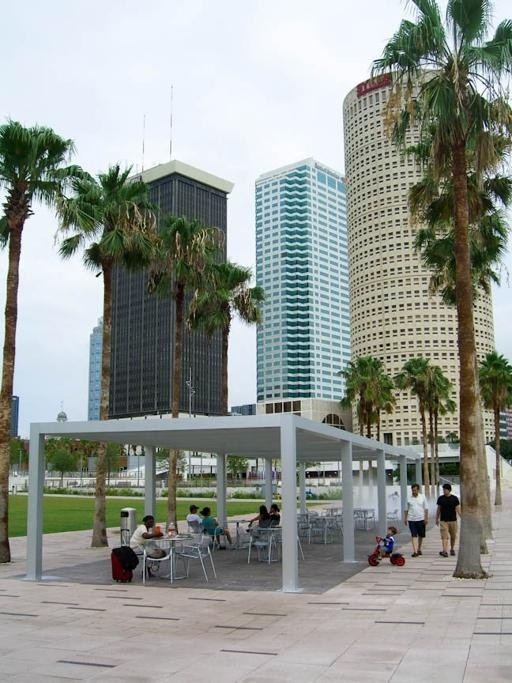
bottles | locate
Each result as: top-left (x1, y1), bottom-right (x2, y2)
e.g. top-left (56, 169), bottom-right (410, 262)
top-left (166, 522), bottom-right (177, 538)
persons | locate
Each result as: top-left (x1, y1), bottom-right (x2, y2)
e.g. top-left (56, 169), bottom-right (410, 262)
top-left (435, 484), bottom-right (461, 557)
top-left (269, 504), bottom-right (280, 528)
top-left (377, 526), bottom-right (397, 560)
top-left (200, 507), bottom-right (237, 550)
top-left (186, 505), bottom-right (214, 549)
top-left (247, 505), bottom-right (270, 533)
top-left (129, 515), bottom-right (163, 577)
top-left (405, 483), bottom-right (429, 557)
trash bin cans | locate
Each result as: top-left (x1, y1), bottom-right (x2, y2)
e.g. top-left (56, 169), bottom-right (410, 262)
top-left (161, 480), bottom-right (166, 488)
top-left (12, 485), bottom-right (17, 495)
top-left (120, 507), bottom-right (137, 546)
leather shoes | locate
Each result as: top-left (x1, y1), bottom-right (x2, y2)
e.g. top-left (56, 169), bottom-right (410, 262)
top-left (451, 549), bottom-right (455, 554)
top-left (440, 551), bottom-right (448, 556)
top-left (418, 550), bottom-right (422, 554)
top-left (412, 552), bottom-right (418, 556)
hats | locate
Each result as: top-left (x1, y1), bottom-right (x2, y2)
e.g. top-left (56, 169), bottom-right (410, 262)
top-left (190, 504), bottom-right (199, 510)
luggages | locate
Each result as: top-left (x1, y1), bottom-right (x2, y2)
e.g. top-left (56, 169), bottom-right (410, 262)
top-left (110, 528), bottom-right (132, 583)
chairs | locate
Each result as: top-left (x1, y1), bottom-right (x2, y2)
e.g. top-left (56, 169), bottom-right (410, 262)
top-left (121, 508), bottom-right (401, 588)
